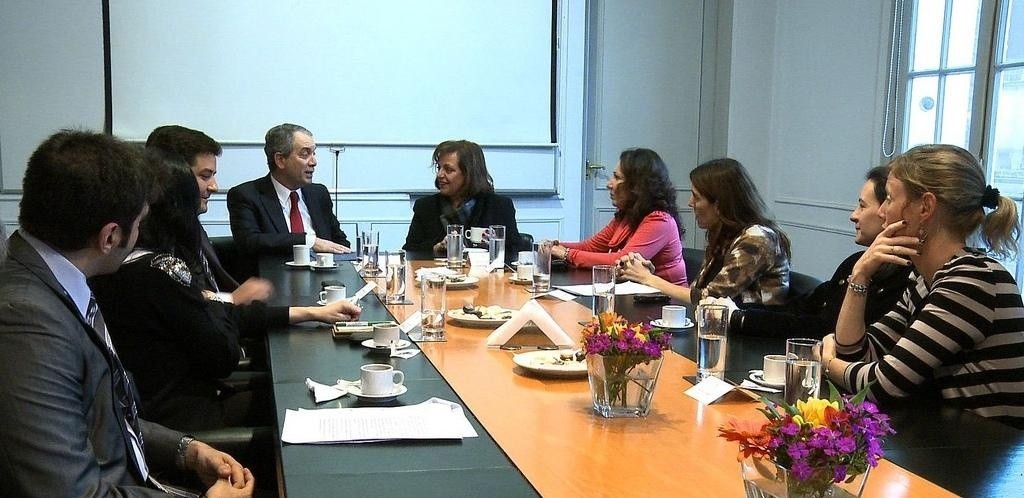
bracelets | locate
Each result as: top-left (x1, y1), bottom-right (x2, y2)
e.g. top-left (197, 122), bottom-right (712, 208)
top-left (846, 275), bottom-right (873, 294)
top-left (563, 247), bottom-right (570, 262)
top-left (730, 309), bottom-right (745, 333)
top-left (175, 435), bottom-right (195, 467)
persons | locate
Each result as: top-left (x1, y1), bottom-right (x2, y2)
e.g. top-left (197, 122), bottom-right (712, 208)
top-left (0, 130), bottom-right (255, 497)
top-left (227, 123), bottom-right (352, 277)
top-left (402, 139), bottom-right (523, 256)
top-left (539, 148), bottom-right (688, 289)
top-left (145, 126), bottom-right (272, 304)
top-left (812, 143), bottom-right (1024, 427)
top-left (694, 167), bottom-right (913, 340)
top-left (610, 158), bottom-right (792, 307)
top-left (97, 149), bottom-right (361, 432)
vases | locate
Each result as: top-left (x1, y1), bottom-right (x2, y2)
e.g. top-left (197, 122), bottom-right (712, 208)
top-left (738, 444), bottom-right (853, 498)
top-left (583, 351), bottom-right (666, 418)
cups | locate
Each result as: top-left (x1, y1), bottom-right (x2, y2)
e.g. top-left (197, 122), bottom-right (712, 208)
top-left (360, 364), bottom-right (404, 396)
top-left (517, 265), bottom-right (533, 280)
top-left (362, 231), bottom-right (379, 272)
top-left (489, 226), bottom-right (505, 269)
top-left (763, 355), bottom-right (786, 385)
top-left (592, 265), bottom-right (616, 319)
top-left (447, 226), bottom-right (463, 268)
top-left (696, 304), bottom-right (729, 383)
top-left (320, 286), bottom-right (346, 303)
top-left (373, 324), bottom-right (400, 346)
top-left (784, 339), bottom-right (824, 404)
top-left (386, 250), bottom-right (405, 302)
top-left (465, 227), bottom-right (488, 243)
top-left (293, 245), bottom-right (310, 264)
top-left (316, 253), bottom-right (334, 267)
top-left (519, 251), bottom-right (538, 264)
top-left (662, 306), bottom-right (690, 327)
top-left (422, 276), bottom-right (446, 341)
top-left (532, 241), bottom-right (551, 292)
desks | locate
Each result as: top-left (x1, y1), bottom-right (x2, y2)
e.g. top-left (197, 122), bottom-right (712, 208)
top-left (257, 258), bottom-right (1024, 497)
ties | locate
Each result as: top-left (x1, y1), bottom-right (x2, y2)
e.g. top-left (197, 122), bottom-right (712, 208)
top-left (288, 190), bottom-right (306, 234)
top-left (84, 289), bottom-right (151, 478)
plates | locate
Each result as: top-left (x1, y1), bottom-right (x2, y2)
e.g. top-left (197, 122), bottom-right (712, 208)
top-left (650, 319), bottom-right (694, 332)
top-left (286, 261), bottom-right (312, 267)
top-left (434, 258), bottom-right (466, 262)
top-left (447, 307), bottom-right (519, 325)
top-left (361, 339), bottom-right (411, 351)
top-left (511, 262), bottom-right (519, 266)
top-left (749, 374), bottom-right (785, 390)
top-left (508, 277), bottom-right (532, 284)
top-left (316, 299), bottom-right (350, 305)
top-left (415, 275), bottom-right (480, 289)
top-left (513, 350), bottom-right (589, 377)
top-left (434, 262), bottom-right (466, 266)
top-left (312, 265), bottom-right (339, 269)
top-left (464, 248), bottom-right (488, 253)
top-left (347, 383), bottom-right (407, 402)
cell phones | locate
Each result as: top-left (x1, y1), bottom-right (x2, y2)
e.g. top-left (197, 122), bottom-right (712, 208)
top-left (633, 293), bottom-right (672, 303)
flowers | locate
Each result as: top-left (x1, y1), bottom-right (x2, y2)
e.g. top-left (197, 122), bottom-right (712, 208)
top-left (580, 313), bottom-right (672, 410)
top-left (719, 377), bottom-right (897, 498)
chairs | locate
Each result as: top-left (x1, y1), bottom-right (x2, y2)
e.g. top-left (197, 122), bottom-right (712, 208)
top-left (205, 237), bottom-right (235, 290)
top-left (681, 248), bottom-right (704, 282)
top-left (789, 268), bottom-right (822, 306)
top-left (518, 233), bottom-right (534, 254)
